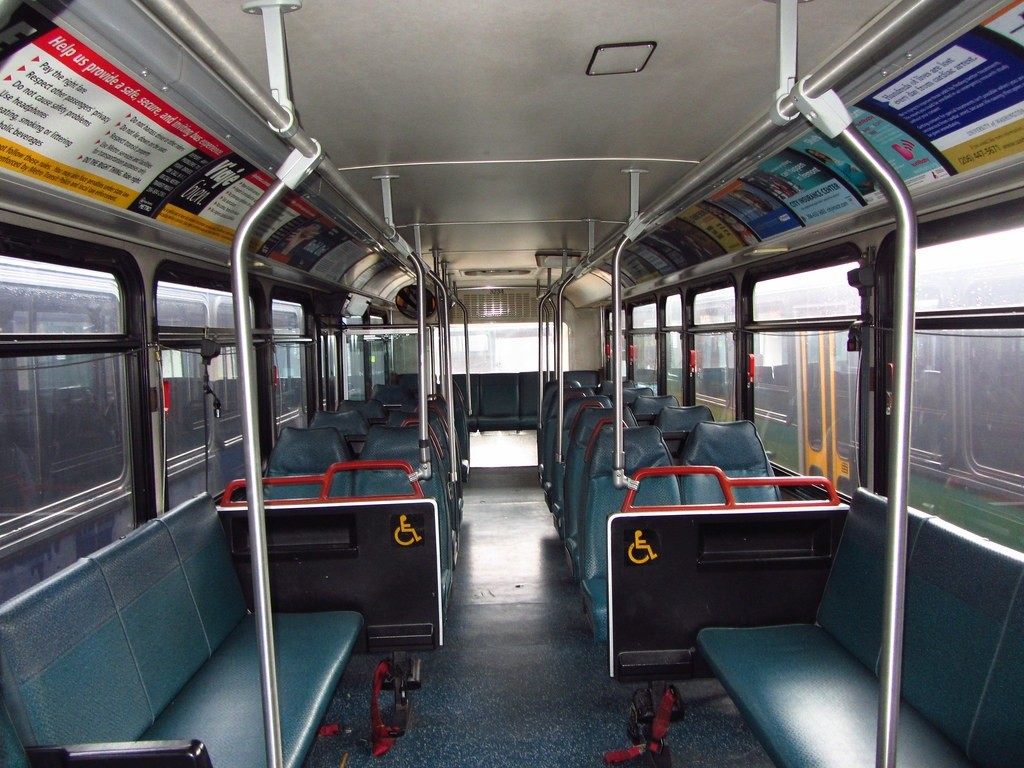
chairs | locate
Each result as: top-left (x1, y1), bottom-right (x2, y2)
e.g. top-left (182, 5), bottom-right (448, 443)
top-left (262, 370), bottom-right (783, 644)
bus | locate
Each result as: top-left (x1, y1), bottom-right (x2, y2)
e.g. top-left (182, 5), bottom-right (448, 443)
top-left (431, 320), bottom-right (569, 375)
top-left (611, 230), bottom-right (1024, 556)
top-left (0, 254), bottom-right (384, 534)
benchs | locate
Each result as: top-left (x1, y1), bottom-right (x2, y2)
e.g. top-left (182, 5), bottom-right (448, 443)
top-left (697, 486), bottom-right (1024, 768)
top-left (0, 490), bottom-right (365, 768)
